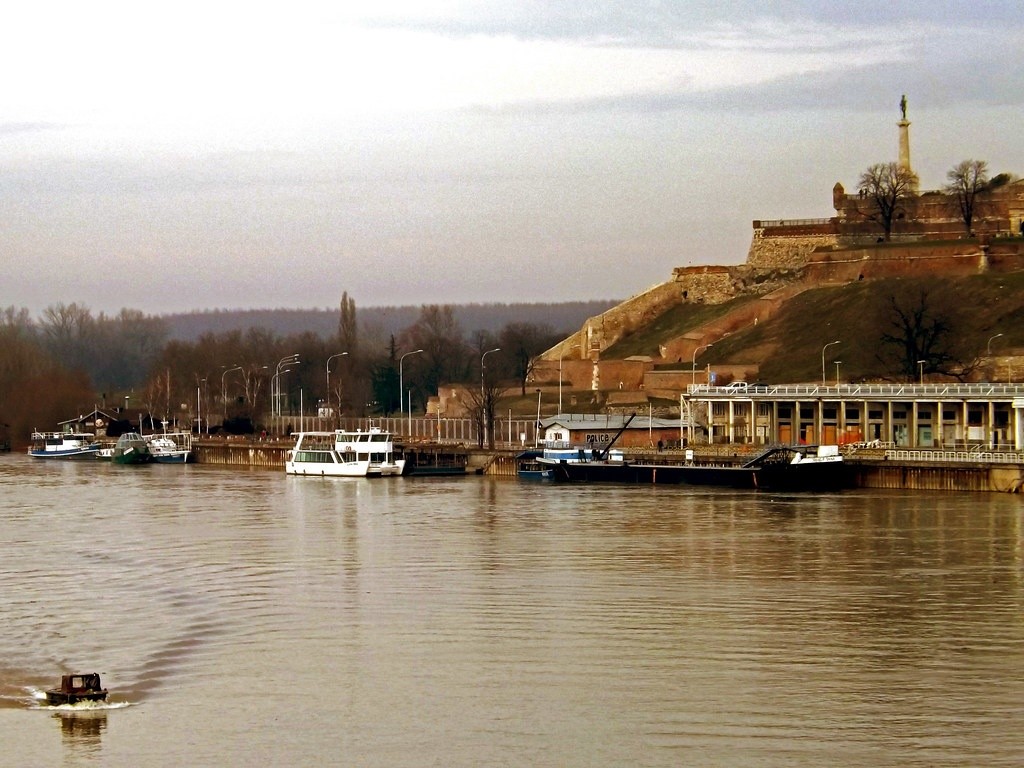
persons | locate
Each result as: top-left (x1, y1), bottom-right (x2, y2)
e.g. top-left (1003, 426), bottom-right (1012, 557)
top-left (658, 438), bottom-right (664, 452)
top-left (286, 421), bottom-right (293, 439)
top-left (261, 430), bottom-right (267, 442)
top-left (588, 438), bottom-right (593, 448)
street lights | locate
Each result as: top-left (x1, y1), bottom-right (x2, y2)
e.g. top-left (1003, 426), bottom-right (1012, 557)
top-left (326, 352), bottom-right (348, 418)
top-left (222, 367), bottom-right (242, 413)
top-left (693, 344), bottom-right (713, 385)
top-left (823, 340), bottom-right (840, 384)
top-left (988, 334), bottom-right (1003, 355)
top-left (262, 354), bottom-right (301, 416)
top-left (482, 348), bottom-right (501, 410)
top-left (400, 350), bottom-right (423, 418)
top-left (559, 344), bottom-right (581, 413)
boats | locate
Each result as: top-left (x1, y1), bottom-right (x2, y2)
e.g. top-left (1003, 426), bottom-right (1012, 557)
top-left (28, 429), bottom-right (99, 457)
top-left (514, 449), bottom-right (623, 482)
top-left (406, 441), bottom-right (469, 476)
top-left (93, 432), bottom-right (193, 462)
top-left (284, 427), bottom-right (406, 477)
